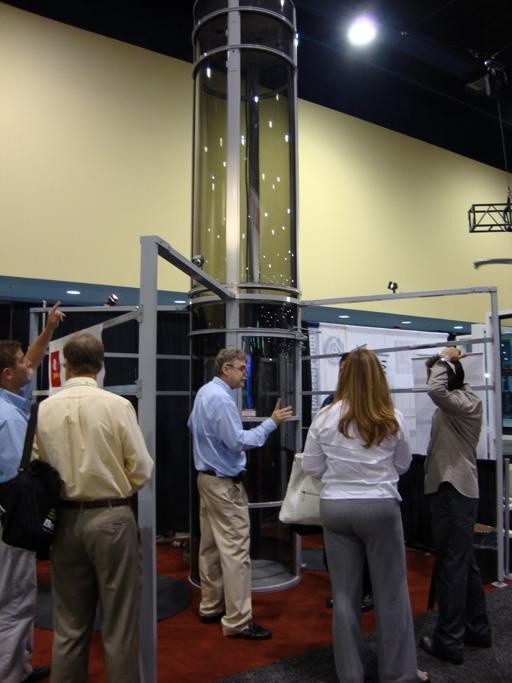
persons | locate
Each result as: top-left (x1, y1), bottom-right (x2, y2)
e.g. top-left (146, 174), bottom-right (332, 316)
top-left (422, 346), bottom-right (491, 665)
top-left (301, 348), bottom-right (431, 683)
top-left (31, 333), bottom-right (155, 683)
top-left (1, 299), bottom-right (65, 683)
top-left (323, 353), bottom-right (375, 612)
top-left (187, 348), bottom-right (294, 640)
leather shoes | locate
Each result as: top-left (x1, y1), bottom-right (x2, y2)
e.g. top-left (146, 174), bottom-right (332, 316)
top-left (326, 597), bottom-right (335, 608)
top-left (225, 620), bottom-right (272, 641)
top-left (362, 593), bottom-right (373, 610)
top-left (23, 666), bottom-right (50, 682)
top-left (422, 636), bottom-right (463, 665)
top-left (199, 611), bottom-right (225, 625)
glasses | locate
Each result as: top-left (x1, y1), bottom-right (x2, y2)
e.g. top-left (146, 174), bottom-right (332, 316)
top-left (229, 365), bottom-right (248, 373)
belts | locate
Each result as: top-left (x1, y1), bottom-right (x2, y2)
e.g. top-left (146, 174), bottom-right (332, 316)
top-left (62, 495), bottom-right (131, 508)
top-left (202, 470), bottom-right (216, 477)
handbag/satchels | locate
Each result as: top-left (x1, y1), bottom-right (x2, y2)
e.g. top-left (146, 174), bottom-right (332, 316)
top-left (0, 458), bottom-right (67, 553)
top-left (278, 452), bottom-right (322, 526)
